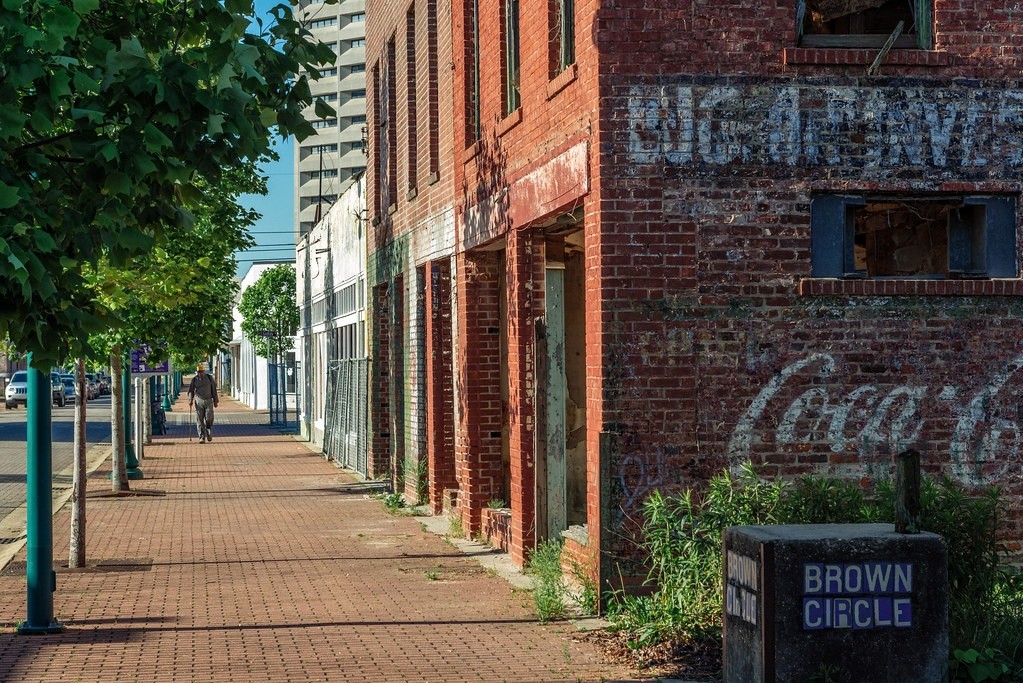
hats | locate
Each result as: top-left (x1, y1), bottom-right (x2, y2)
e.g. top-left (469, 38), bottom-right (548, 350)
top-left (196, 366), bottom-right (205, 372)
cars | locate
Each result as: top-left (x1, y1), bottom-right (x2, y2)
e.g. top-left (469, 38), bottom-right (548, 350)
top-left (5, 370), bottom-right (111, 409)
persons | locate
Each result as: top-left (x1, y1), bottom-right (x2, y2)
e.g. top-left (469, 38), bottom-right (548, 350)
top-left (188, 365), bottom-right (219, 443)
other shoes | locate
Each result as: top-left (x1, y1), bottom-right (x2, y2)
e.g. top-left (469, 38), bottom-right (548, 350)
top-left (207, 433), bottom-right (212, 441)
top-left (200, 437), bottom-right (205, 443)
